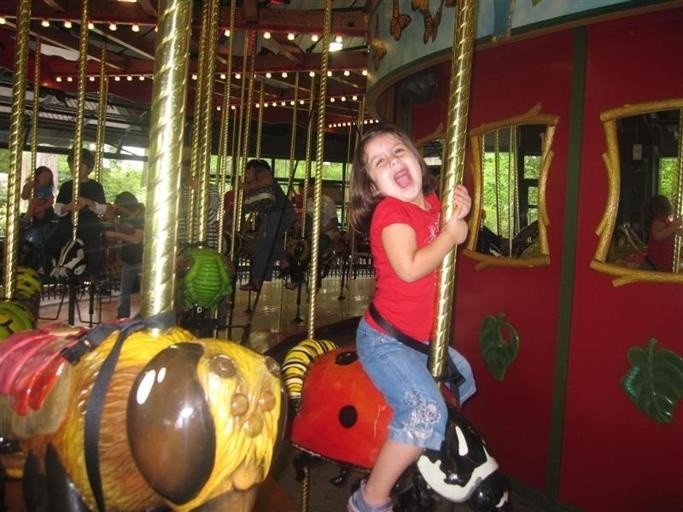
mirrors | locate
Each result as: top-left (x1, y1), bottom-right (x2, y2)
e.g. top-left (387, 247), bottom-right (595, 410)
top-left (590, 97), bottom-right (683, 286)
top-left (462, 104), bottom-right (555, 271)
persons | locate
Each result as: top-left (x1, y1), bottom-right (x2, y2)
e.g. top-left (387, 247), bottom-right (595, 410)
top-left (179, 158), bottom-right (338, 292)
top-left (473, 207), bottom-right (511, 259)
top-left (347, 122), bottom-right (479, 509)
top-left (642, 192), bottom-right (682, 273)
top-left (19, 147), bottom-right (145, 319)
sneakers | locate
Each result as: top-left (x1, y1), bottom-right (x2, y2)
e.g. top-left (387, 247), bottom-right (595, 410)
top-left (345, 483), bottom-right (396, 512)
